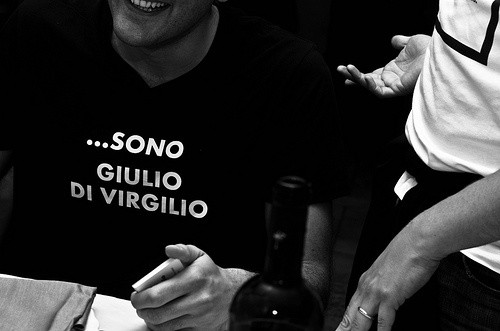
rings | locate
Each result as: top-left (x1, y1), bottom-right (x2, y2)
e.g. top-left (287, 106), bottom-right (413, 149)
top-left (357, 306), bottom-right (374, 320)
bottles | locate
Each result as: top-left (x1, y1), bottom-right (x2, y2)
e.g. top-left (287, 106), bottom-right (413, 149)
top-left (228, 176), bottom-right (324, 331)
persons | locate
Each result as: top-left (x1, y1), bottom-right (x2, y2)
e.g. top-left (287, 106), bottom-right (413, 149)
top-left (335, 0), bottom-right (500, 331)
top-left (0, 0), bottom-right (357, 331)
